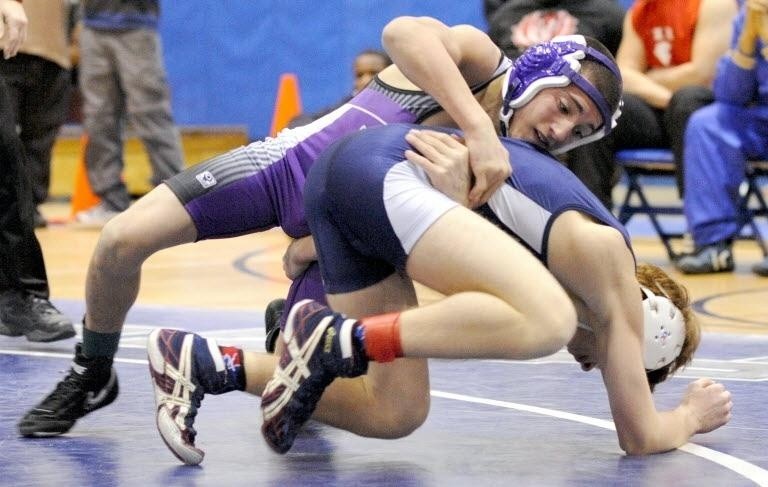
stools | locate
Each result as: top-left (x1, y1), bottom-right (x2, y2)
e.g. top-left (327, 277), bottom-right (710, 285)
top-left (617, 150), bottom-right (685, 258)
top-left (732, 162), bottom-right (768, 257)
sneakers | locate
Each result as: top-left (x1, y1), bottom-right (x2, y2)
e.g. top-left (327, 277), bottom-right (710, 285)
top-left (14, 341), bottom-right (121, 441)
top-left (259, 297), bottom-right (370, 457)
top-left (0, 287), bottom-right (77, 343)
top-left (750, 257), bottom-right (768, 280)
top-left (75, 202), bottom-right (125, 231)
top-left (676, 242), bottom-right (735, 274)
top-left (144, 324), bottom-right (237, 468)
top-left (265, 297), bottom-right (289, 354)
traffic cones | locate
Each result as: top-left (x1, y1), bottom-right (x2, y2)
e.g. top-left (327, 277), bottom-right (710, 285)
top-left (270, 72), bottom-right (303, 139)
top-left (68, 129), bottom-right (127, 227)
